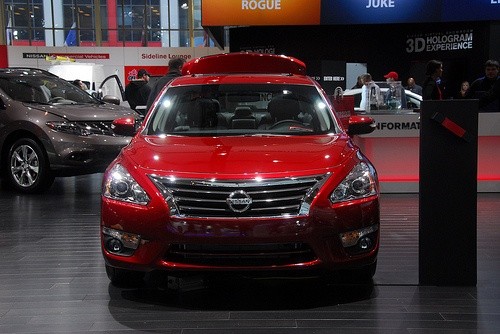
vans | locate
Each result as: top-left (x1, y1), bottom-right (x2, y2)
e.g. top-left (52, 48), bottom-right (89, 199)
top-left (49, 61), bottom-right (105, 99)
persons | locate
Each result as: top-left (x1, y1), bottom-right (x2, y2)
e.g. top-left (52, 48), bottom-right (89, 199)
top-left (452, 81), bottom-right (470, 99)
top-left (464, 59), bottom-right (500, 113)
top-left (404, 77), bottom-right (423, 96)
top-left (145, 58), bottom-right (186, 113)
top-left (125, 69), bottom-right (153, 122)
top-left (340, 72), bottom-right (381, 113)
top-left (352, 76), bottom-right (364, 107)
top-left (377, 71), bottom-right (408, 111)
top-left (422, 60), bottom-right (449, 101)
top-left (65, 79), bottom-right (85, 102)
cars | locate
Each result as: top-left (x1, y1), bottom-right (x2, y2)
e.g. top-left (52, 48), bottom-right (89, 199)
top-left (333, 86), bottom-right (424, 114)
top-left (101, 51), bottom-right (382, 287)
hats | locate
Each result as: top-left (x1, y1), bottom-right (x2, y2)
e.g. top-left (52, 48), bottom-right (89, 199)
top-left (138, 69), bottom-right (151, 76)
top-left (384, 72), bottom-right (398, 80)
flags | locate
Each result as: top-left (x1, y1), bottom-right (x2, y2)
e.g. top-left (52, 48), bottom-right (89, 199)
top-left (64, 22), bottom-right (77, 46)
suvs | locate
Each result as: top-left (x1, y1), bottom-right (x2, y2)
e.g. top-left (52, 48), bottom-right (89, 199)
top-left (0, 65), bottom-right (148, 194)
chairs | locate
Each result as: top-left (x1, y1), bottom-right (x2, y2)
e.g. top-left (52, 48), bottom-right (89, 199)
top-left (181, 99), bottom-right (227, 130)
top-left (267, 95), bottom-right (301, 121)
